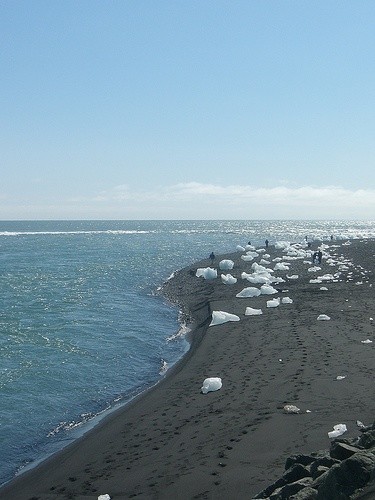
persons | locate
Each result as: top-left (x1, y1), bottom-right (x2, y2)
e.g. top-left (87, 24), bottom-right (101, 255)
top-left (318, 251), bottom-right (322, 264)
top-left (210, 252), bottom-right (215, 264)
top-left (248, 242), bottom-right (251, 245)
top-left (265, 240), bottom-right (269, 247)
top-left (312, 252), bottom-right (317, 263)
